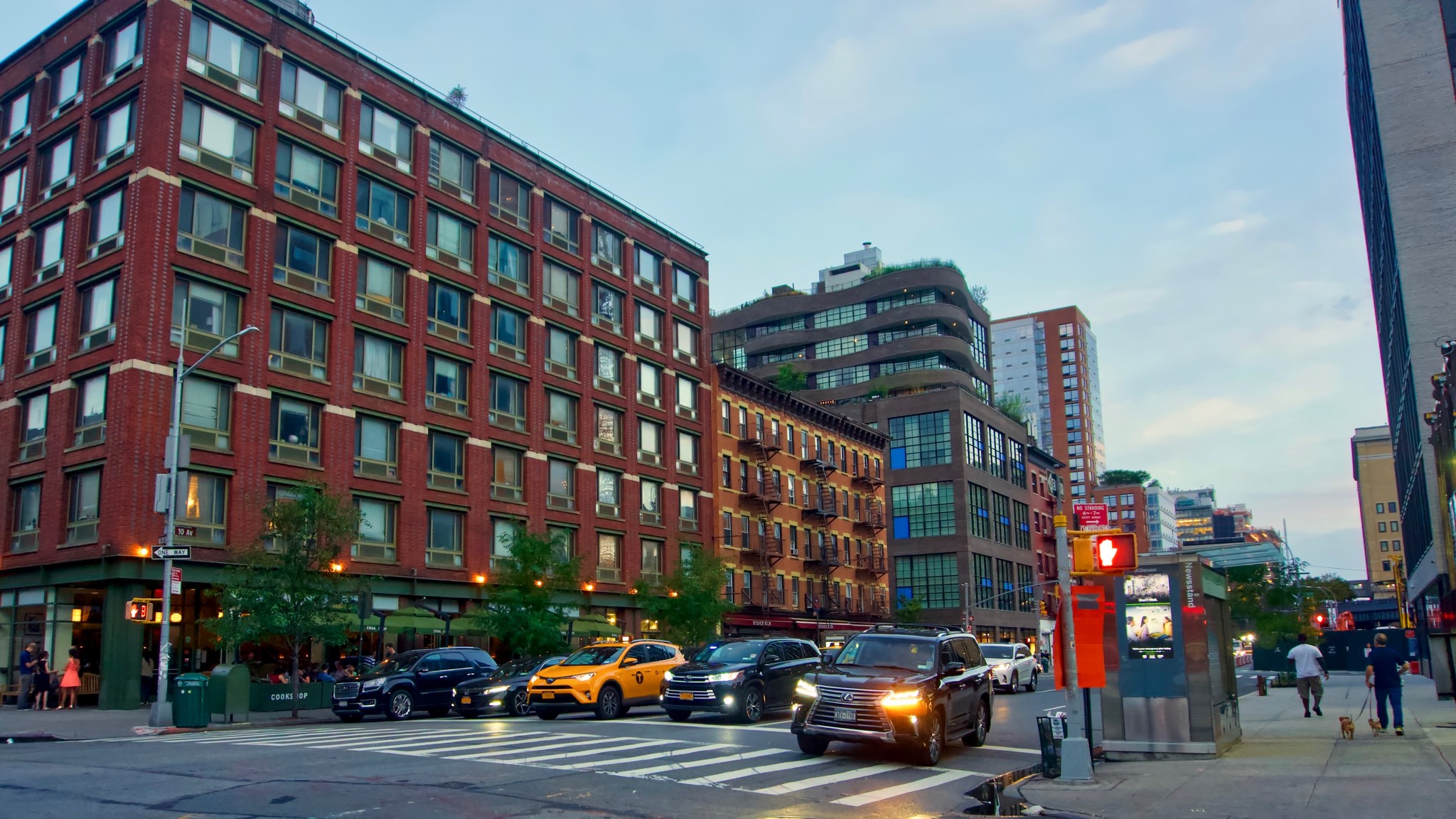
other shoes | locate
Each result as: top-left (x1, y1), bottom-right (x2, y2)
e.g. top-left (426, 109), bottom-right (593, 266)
top-left (1313, 707), bottom-right (1323, 716)
top-left (56, 706), bottom-right (64, 709)
top-left (1395, 726), bottom-right (1404, 736)
top-left (67, 705), bottom-right (73, 709)
top-left (17, 707), bottom-right (30, 711)
top-left (42, 707), bottom-right (51, 710)
top-left (1304, 711), bottom-right (1311, 717)
top-left (1381, 727), bottom-right (1387, 732)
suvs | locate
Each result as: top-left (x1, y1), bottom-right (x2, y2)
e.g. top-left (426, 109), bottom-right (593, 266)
top-left (527, 638), bottom-right (689, 721)
top-left (979, 642), bottom-right (1037, 694)
top-left (332, 645), bottom-right (505, 723)
top-left (790, 622), bottom-right (995, 766)
top-left (659, 636), bottom-right (859, 724)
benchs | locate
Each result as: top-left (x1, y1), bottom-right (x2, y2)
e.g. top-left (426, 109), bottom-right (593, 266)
top-left (56, 673), bottom-right (100, 705)
top-left (0, 677), bottom-right (34, 708)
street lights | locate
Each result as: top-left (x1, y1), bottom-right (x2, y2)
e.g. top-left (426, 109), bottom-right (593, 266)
top-left (1246, 529), bottom-right (1338, 634)
top-left (148, 299), bottom-right (260, 727)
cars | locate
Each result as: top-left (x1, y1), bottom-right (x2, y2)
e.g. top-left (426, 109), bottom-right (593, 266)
top-left (451, 655), bottom-right (590, 718)
top-left (1233, 640), bottom-right (1253, 654)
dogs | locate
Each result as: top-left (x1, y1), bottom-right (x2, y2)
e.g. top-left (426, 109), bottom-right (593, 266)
top-left (1368, 718), bottom-right (1382, 737)
top-left (1339, 714), bottom-right (1354, 740)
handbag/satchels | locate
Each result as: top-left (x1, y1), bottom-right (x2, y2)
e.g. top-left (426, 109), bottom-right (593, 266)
top-left (49, 674), bottom-right (59, 687)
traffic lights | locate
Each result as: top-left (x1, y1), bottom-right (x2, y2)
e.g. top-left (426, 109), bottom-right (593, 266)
top-left (1039, 602), bottom-right (1047, 617)
top-left (129, 601), bottom-right (146, 621)
top-left (1096, 532), bottom-right (1138, 570)
top-left (1027, 637), bottom-right (1034, 643)
top-left (1317, 616), bottom-right (1324, 623)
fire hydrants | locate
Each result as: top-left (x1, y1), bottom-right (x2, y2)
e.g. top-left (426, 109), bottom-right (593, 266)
top-left (1257, 676), bottom-right (1268, 696)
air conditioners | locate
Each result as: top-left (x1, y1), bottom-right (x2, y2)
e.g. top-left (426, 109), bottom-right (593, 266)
top-left (791, 549), bottom-right (798, 555)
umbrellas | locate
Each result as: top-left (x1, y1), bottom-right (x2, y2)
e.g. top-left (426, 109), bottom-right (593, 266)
top-left (232, 602), bottom-right (635, 665)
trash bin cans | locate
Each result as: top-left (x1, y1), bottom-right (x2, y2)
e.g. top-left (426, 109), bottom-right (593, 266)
top-left (1036, 712), bottom-right (1068, 777)
top-left (172, 672), bottom-right (210, 728)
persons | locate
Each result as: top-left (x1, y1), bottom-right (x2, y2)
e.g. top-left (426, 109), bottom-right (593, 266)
top-left (16, 643), bottom-right (90, 711)
top-left (240, 647), bottom-right (377, 685)
top-left (1365, 632), bottom-right (1408, 736)
top-left (1127, 616), bottom-right (1173, 648)
top-left (198, 647), bottom-right (213, 672)
top-left (140, 650), bottom-right (154, 705)
top-left (385, 643), bottom-right (399, 659)
top-left (1034, 649), bottom-right (1051, 661)
top-left (1287, 634), bottom-right (1329, 718)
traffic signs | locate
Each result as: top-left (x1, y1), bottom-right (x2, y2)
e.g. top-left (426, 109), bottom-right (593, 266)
top-left (1075, 504), bottom-right (1108, 527)
top-left (1047, 469), bottom-right (1065, 501)
top-left (151, 546), bottom-right (191, 560)
